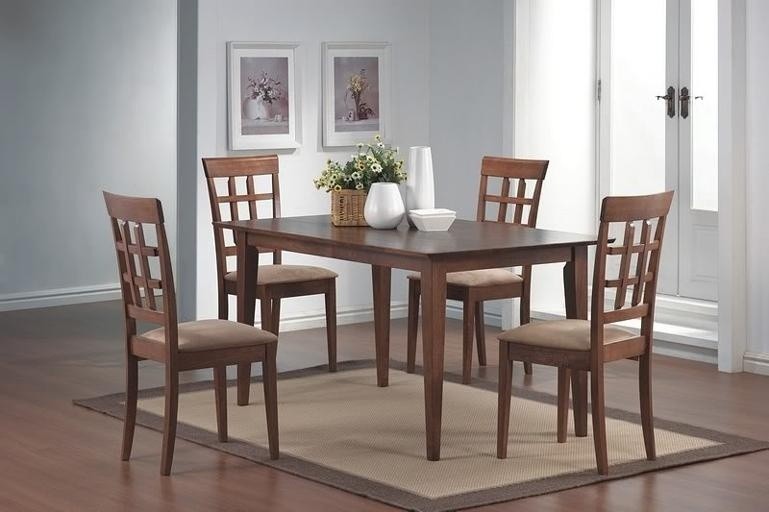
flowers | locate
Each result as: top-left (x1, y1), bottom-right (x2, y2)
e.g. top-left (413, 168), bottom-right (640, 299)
top-left (313, 135), bottom-right (408, 193)
top-left (246, 71), bottom-right (284, 103)
top-left (342, 71), bottom-right (375, 121)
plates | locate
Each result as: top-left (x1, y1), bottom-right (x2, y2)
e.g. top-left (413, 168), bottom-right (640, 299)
top-left (410, 208), bottom-right (455, 233)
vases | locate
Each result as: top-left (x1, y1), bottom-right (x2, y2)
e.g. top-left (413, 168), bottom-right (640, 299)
top-left (244, 98), bottom-right (268, 121)
top-left (364, 182), bottom-right (406, 230)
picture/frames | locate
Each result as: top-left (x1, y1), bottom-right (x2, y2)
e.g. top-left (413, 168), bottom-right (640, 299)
top-left (226, 40), bottom-right (296, 151)
top-left (321, 41), bottom-right (392, 146)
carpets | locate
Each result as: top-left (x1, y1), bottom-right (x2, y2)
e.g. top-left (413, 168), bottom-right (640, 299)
top-left (72, 359), bottom-right (768, 512)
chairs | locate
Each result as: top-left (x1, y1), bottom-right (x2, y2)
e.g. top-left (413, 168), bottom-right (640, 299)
top-left (407, 155), bottom-right (549, 385)
top-left (202, 154), bottom-right (338, 373)
top-left (497, 190), bottom-right (675, 475)
top-left (102, 190), bottom-right (279, 477)
